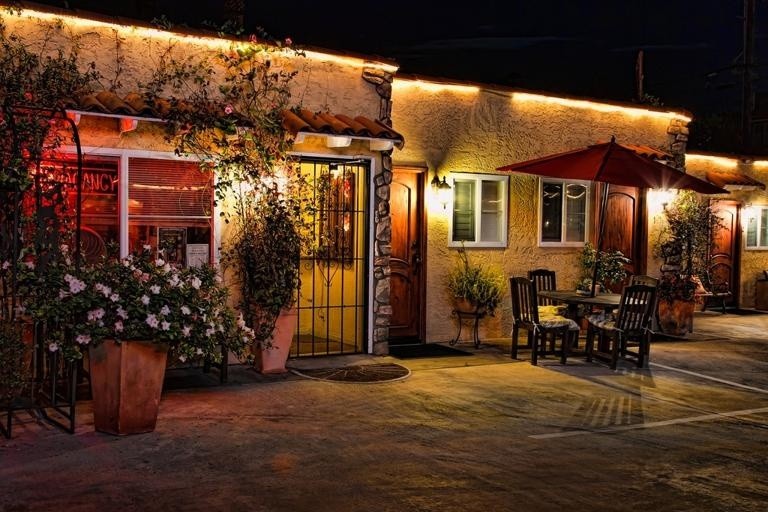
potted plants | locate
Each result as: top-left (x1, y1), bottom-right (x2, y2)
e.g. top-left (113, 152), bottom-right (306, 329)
top-left (441, 238), bottom-right (508, 319)
top-left (575, 240), bottom-right (633, 330)
top-left (654, 191), bottom-right (728, 337)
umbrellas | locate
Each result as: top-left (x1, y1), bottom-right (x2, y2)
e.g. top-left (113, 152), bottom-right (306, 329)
top-left (492, 134), bottom-right (730, 315)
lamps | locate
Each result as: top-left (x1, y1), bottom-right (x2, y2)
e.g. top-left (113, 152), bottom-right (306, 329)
top-left (431, 175), bottom-right (452, 208)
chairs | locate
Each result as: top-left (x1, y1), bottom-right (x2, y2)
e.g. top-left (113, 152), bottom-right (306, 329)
top-left (508, 269), bottom-right (661, 370)
top-left (700, 270), bottom-right (732, 314)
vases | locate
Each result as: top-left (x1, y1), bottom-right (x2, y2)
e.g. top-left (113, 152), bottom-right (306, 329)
top-left (88, 339), bottom-right (170, 436)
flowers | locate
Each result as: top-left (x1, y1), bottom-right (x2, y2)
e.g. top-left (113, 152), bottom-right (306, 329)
top-left (3, 232), bottom-right (256, 368)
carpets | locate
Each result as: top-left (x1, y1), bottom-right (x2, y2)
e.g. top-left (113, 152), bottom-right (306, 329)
top-left (385, 343), bottom-right (475, 360)
